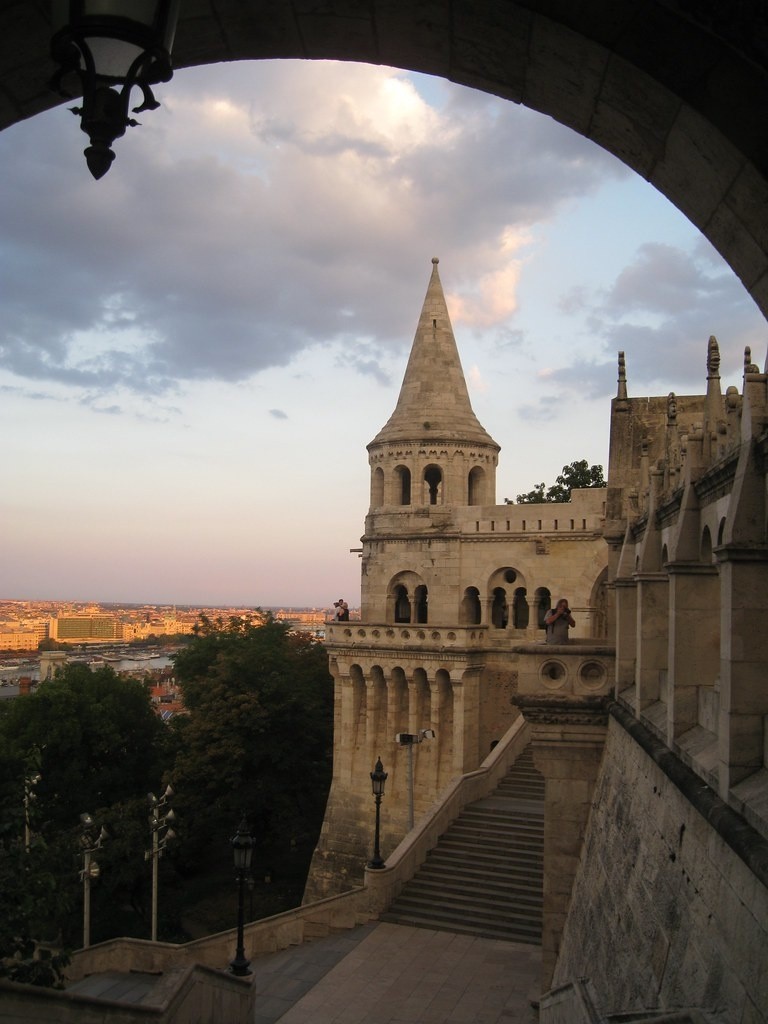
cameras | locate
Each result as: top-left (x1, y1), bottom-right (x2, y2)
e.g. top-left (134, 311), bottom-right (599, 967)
top-left (564, 608), bottom-right (571, 614)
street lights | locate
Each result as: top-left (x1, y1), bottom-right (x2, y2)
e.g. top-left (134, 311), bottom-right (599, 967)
top-left (23, 774), bottom-right (41, 853)
top-left (144, 784), bottom-right (178, 942)
top-left (396, 728), bottom-right (435, 831)
top-left (368, 756), bottom-right (388, 869)
top-left (77, 812), bottom-right (113, 947)
top-left (228, 816), bottom-right (256, 977)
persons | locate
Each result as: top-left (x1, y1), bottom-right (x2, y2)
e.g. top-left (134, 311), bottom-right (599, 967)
top-left (331, 599), bottom-right (349, 621)
top-left (544, 599), bottom-right (576, 645)
